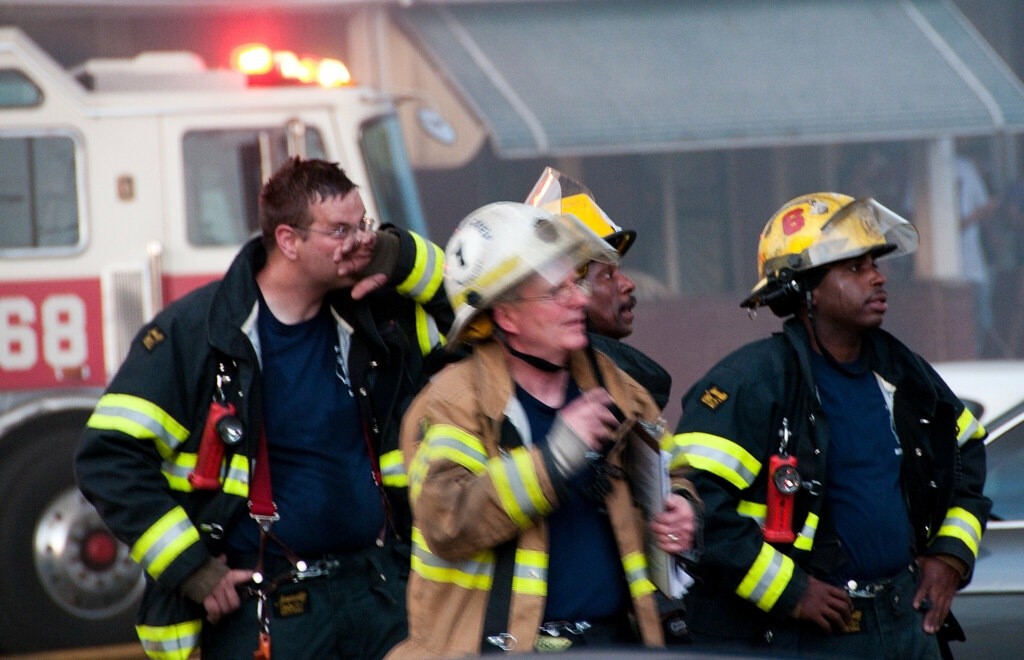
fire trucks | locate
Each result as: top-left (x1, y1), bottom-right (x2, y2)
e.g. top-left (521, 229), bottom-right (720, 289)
top-left (0, 19), bottom-right (459, 656)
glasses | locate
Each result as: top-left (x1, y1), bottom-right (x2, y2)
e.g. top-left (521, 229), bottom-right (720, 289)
top-left (293, 216), bottom-right (375, 241)
top-left (499, 276), bottom-right (595, 305)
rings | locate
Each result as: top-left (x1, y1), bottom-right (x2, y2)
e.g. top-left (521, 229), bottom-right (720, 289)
top-left (668, 534), bottom-right (679, 541)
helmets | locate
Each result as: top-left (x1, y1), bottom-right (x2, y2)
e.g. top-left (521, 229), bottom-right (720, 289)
top-left (523, 165), bottom-right (636, 261)
top-left (441, 202), bottom-right (613, 342)
top-left (740, 190), bottom-right (919, 312)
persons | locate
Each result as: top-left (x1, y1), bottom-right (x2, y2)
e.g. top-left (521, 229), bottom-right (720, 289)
top-left (523, 166), bottom-right (672, 413)
top-left (384, 201), bottom-right (704, 659)
top-left (672, 191), bottom-right (994, 660)
top-left (77, 153), bottom-right (454, 658)
